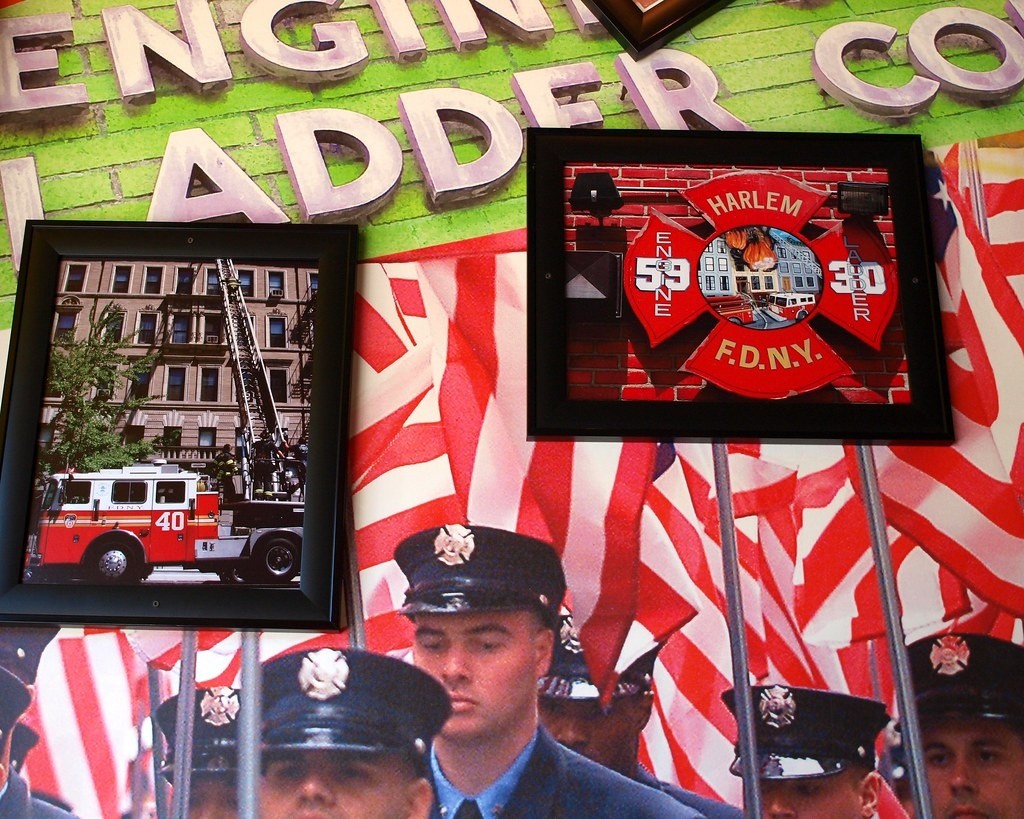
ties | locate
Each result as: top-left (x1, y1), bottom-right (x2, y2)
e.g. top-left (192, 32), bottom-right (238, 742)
top-left (453, 800), bottom-right (483, 819)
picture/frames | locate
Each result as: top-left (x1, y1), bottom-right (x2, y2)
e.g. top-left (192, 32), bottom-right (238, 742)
top-left (525, 127), bottom-right (955, 447)
top-left (582, 0), bottom-right (735, 63)
top-left (0, 217), bottom-right (358, 632)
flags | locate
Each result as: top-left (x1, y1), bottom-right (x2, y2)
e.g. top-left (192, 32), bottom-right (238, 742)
top-left (16, 131), bottom-right (1024, 818)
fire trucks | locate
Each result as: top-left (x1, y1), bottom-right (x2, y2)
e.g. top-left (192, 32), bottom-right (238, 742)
top-left (29, 258), bottom-right (310, 588)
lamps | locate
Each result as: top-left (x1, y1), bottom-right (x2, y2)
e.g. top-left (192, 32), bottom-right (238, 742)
top-left (838, 178), bottom-right (890, 215)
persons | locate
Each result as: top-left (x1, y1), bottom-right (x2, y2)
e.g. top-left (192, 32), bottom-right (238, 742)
top-left (155, 687), bottom-right (241, 819)
top-left (537, 615), bottom-right (743, 819)
top-left (720, 683), bottom-right (891, 818)
top-left (394, 524), bottom-right (709, 819)
top-left (209, 444), bottom-right (240, 497)
top-left (252, 430), bottom-right (285, 500)
top-left (281, 437), bottom-right (308, 502)
top-left (260, 647), bottom-right (452, 818)
top-left (877, 633), bottom-right (1024, 819)
top-left (0, 627), bottom-right (81, 818)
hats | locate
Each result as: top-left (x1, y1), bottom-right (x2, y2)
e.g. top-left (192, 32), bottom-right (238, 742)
top-left (0, 619), bottom-right (62, 713)
top-left (391, 525), bottom-right (567, 629)
top-left (156, 686), bottom-right (247, 788)
top-left (256, 649), bottom-right (452, 763)
top-left (721, 685), bottom-right (890, 780)
top-left (536, 606), bottom-right (659, 699)
top-left (903, 632), bottom-right (1024, 726)
